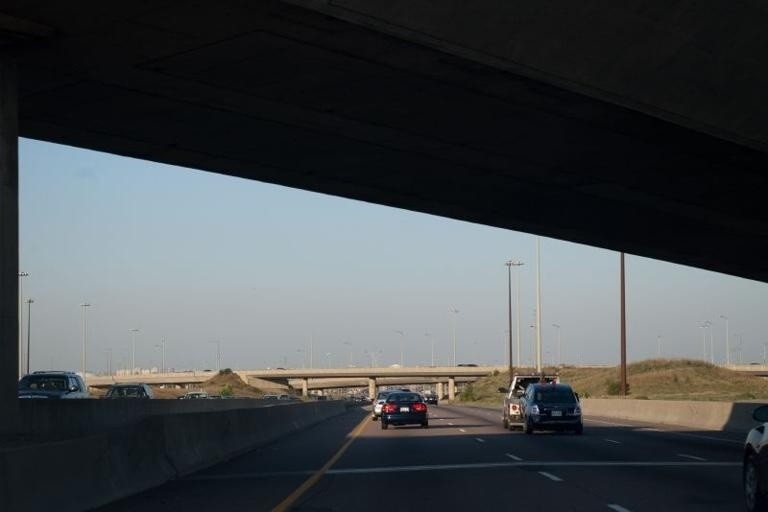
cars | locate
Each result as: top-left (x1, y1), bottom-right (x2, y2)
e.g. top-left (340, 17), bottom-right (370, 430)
top-left (371, 389), bottom-right (437, 429)
top-left (262, 394), bottom-right (289, 400)
top-left (178, 392), bottom-right (209, 399)
top-left (100, 384), bottom-right (154, 399)
top-left (496, 375), bottom-right (582, 434)
top-left (743, 405), bottom-right (768, 512)
top-left (19, 371), bottom-right (88, 399)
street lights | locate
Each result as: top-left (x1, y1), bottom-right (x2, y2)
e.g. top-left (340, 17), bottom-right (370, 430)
top-left (80, 302), bottom-right (92, 389)
top-left (700, 315), bottom-right (730, 363)
top-left (128, 329), bottom-right (137, 375)
top-left (19, 271), bottom-right (34, 380)
top-left (513, 261), bottom-right (525, 368)
top-left (449, 310), bottom-right (458, 367)
top-left (505, 261), bottom-right (514, 387)
top-left (425, 332), bottom-right (433, 366)
top-left (552, 323), bottom-right (561, 363)
